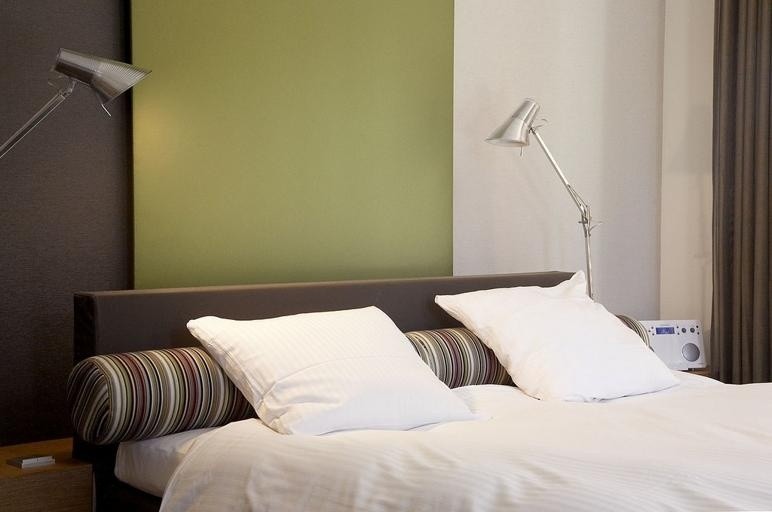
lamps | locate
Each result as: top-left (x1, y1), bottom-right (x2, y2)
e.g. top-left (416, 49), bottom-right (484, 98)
top-left (0, 46), bottom-right (152, 169)
top-left (483, 97), bottom-right (595, 298)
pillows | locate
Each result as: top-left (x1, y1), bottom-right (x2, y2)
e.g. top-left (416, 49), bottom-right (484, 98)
top-left (65, 341), bottom-right (256, 447)
top-left (184, 305), bottom-right (470, 438)
top-left (402, 315), bottom-right (652, 390)
top-left (434, 272), bottom-right (679, 404)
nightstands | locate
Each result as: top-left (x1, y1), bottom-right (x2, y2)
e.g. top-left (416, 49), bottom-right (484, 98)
top-left (0, 435), bottom-right (92, 512)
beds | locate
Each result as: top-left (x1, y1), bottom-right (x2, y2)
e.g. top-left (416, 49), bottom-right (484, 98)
top-left (75, 274), bottom-right (772, 512)
